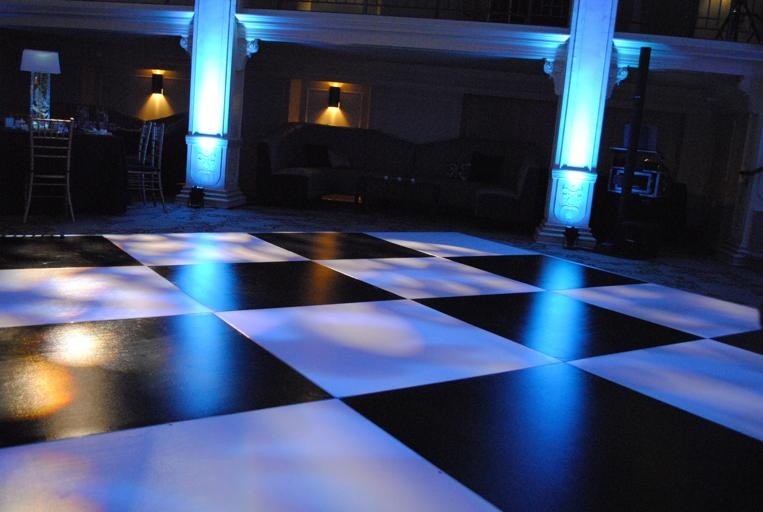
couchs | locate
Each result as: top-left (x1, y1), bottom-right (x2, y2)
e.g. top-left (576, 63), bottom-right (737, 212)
top-left (256, 121), bottom-right (553, 222)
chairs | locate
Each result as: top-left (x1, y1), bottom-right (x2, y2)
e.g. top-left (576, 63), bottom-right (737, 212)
top-left (22, 116), bottom-right (169, 226)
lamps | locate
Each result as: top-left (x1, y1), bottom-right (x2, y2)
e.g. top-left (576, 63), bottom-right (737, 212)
top-left (18, 48), bottom-right (62, 126)
top-left (330, 86), bottom-right (339, 106)
top-left (152, 73), bottom-right (162, 94)
